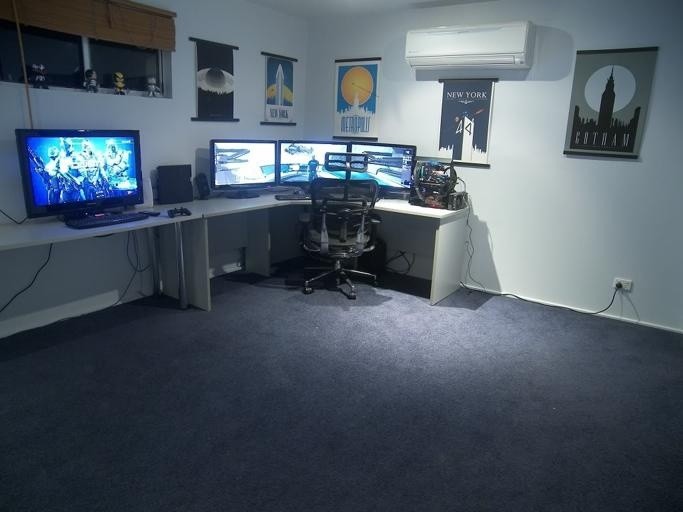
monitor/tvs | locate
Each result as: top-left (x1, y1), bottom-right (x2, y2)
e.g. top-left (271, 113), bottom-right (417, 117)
top-left (280, 139), bottom-right (350, 192)
top-left (209, 137), bottom-right (279, 199)
top-left (14, 128), bottom-right (144, 218)
top-left (350, 141), bottom-right (417, 193)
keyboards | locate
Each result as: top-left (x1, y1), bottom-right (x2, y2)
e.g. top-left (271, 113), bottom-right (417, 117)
top-left (64, 210), bottom-right (150, 229)
top-left (276, 192), bottom-right (313, 201)
top-left (379, 191), bottom-right (413, 200)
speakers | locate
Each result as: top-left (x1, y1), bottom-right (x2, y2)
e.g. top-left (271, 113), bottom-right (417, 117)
top-left (157, 164), bottom-right (194, 205)
top-left (195, 172), bottom-right (211, 198)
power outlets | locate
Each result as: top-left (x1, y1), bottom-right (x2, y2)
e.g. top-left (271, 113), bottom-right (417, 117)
top-left (613, 277), bottom-right (633, 292)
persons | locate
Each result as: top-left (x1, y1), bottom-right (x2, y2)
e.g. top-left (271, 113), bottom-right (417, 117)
top-left (308, 155), bottom-right (319, 181)
top-left (33, 138), bottom-right (132, 205)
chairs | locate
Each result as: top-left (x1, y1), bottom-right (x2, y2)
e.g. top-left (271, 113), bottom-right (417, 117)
top-left (302, 152), bottom-right (381, 300)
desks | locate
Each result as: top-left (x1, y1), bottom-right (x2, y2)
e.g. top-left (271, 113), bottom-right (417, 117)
top-left (212, 191), bottom-right (469, 304)
top-left (2, 201), bottom-right (210, 337)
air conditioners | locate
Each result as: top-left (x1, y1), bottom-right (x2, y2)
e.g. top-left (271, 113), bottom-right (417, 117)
top-left (405, 19), bottom-right (536, 69)
